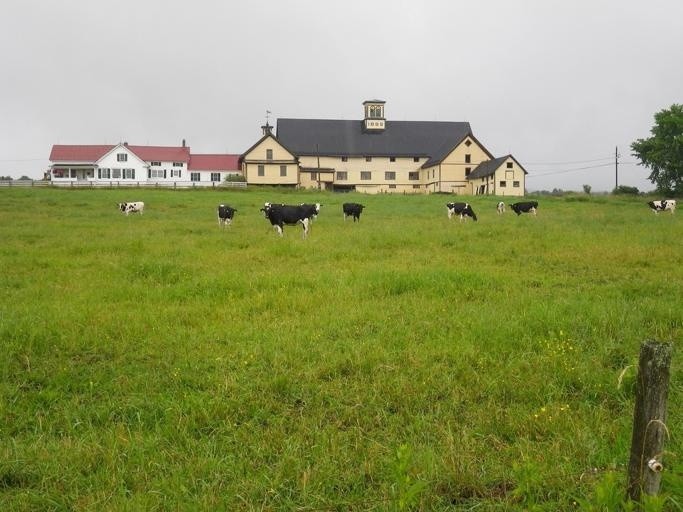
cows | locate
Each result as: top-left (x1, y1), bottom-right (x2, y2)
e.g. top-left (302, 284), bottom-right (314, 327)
top-left (508, 201), bottom-right (537, 216)
top-left (342, 203), bottom-right (365, 223)
top-left (646, 199), bottom-right (675, 215)
top-left (116, 201), bottom-right (143, 216)
top-left (260, 202), bottom-right (323, 239)
top-left (497, 202), bottom-right (504, 214)
top-left (446, 201), bottom-right (476, 223)
top-left (216, 205), bottom-right (237, 226)
